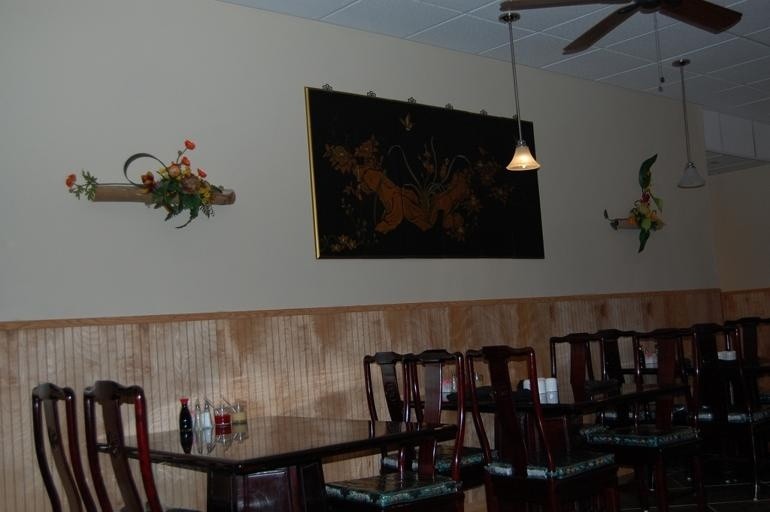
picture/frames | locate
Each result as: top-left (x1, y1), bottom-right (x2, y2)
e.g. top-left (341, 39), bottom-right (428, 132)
top-left (303, 84), bottom-right (546, 261)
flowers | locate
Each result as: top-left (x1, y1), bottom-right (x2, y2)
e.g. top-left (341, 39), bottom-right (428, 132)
top-left (125, 136), bottom-right (220, 229)
top-left (61, 170), bottom-right (100, 201)
top-left (604, 153), bottom-right (667, 254)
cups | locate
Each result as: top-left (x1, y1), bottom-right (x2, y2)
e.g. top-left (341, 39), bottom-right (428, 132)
top-left (717, 351), bottom-right (737, 361)
top-left (523, 377), bottom-right (558, 395)
top-left (539, 392), bottom-right (559, 404)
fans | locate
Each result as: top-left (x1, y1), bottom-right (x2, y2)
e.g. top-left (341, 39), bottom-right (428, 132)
top-left (498, 1), bottom-right (741, 91)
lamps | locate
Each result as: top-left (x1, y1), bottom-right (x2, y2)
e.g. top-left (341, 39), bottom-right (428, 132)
top-left (671, 57), bottom-right (706, 190)
top-left (500, 11), bottom-right (542, 172)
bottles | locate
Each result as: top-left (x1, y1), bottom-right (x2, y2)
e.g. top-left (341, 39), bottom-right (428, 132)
top-left (639, 344), bottom-right (646, 370)
top-left (179, 398), bottom-right (193, 432)
top-left (232, 424), bottom-right (250, 444)
top-left (202, 403), bottom-right (214, 429)
top-left (230, 401), bottom-right (248, 424)
top-left (180, 431), bottom-right (194, 455)
top-left (213, 404), bottom-right (232, 427)
top-left (196, 432), bottom-right (204, 455)
top-left (204, 428), bottom-right (214, 453)
top-left (215, 424), bottom-right (231, 446)
top-left (193, 404), bottom-right (204, 433)
